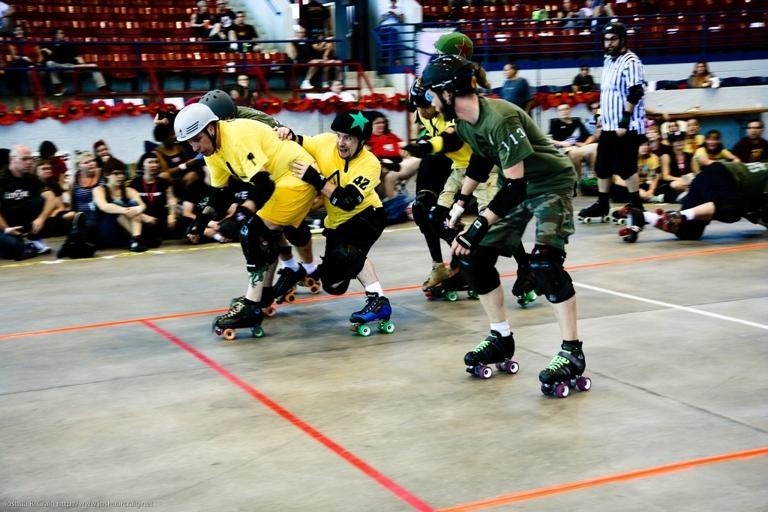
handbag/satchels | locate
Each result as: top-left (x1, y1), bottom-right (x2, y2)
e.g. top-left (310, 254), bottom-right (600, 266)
top-left (59, 233), bottom-right (93, 258)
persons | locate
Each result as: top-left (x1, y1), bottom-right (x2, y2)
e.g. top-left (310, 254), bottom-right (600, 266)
top-left (402, 32), bottom-right (538, 306)
top-left (1, 0), bottom-right (767, 259)
top-left (173, 91), bottom-right (395, 339)
top-left (417, 53), bottom-right (592, 397)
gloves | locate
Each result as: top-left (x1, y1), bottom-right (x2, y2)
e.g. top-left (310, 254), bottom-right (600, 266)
top-left (457, 216), bottom-right (492, 250)
top-left (618, 111), bottom-right (632, 131)
top-left (302, 165), bottom-right (328, 190)
top-left (445, 189), bottom-right (473, 229)
top-left (401, 140), bottom-right (433, 158)
top-left (186, 211), bottom-right (211, 235)
top-left (219, 206), bottom-right (251, 238)
top-left (288, 129), bottom-right (304, 146)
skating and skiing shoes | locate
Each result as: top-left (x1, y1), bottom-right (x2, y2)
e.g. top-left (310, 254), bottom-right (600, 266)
top-left (275, 264), bottom-right (321, 302)
top-left (512, 257), bottom-right (544, 306)
top-left (539, 341), bottom-right (591, 397)
top-left (215, 298), bottom-right (264, 340)
top-left (655, 208), bottom-right (689, 236)
top-left (350, 291), bottom-right (395, 336)
top-left (231, 288), bottom-right (278, 317)
top-left (464, 329), bottom-right (520, 379)
top-left (579, 202), bottom-right (610, 223)
top-left (613, 202), bottom-right (647, 224)
top-left (619, 207), bottom-right (645, 242)
top-left (442, 272), bottom-right (478, 301)
top-left (422, 263), bottom-right (451, 299)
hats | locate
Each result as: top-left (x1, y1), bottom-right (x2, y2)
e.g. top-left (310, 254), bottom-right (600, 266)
top-left (216, 0), bottom-right (226, 5)
top-left (668, 131), bottom-right (686, 143)
top-left (197, 1), bottom-right (208, 7)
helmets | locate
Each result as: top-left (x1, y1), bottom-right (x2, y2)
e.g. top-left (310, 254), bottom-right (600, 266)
top-left (422, 55), bottom-right (473, 91)
top-left (199, 90), bottom-right (238, 119)
top-left (433, 33), bottom-right (475, 59)
top-left (332, 110), bottom-right (374, 141)
top-left (174, 103), bottom-right (219, 143)
top-left (601, 23), bottom-right (628, 39)
top-left (154, 123), bottom-right (174, 141)
top-left (409, 76), bottom-right (433, 104)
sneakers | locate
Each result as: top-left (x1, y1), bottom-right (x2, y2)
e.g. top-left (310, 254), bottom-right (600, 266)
top-left (24, 241), bottom-right (51, 255)
top-left (300, 81), bottom-right (315, 90)
top-left (54, 86), bottom-right (68, 96)
top-left (129, 236), bottom-right (158, 252)
top-left (99, 87), bottom-right (116, 95)
top-left (654, 194), bottom-right (665, 203)
